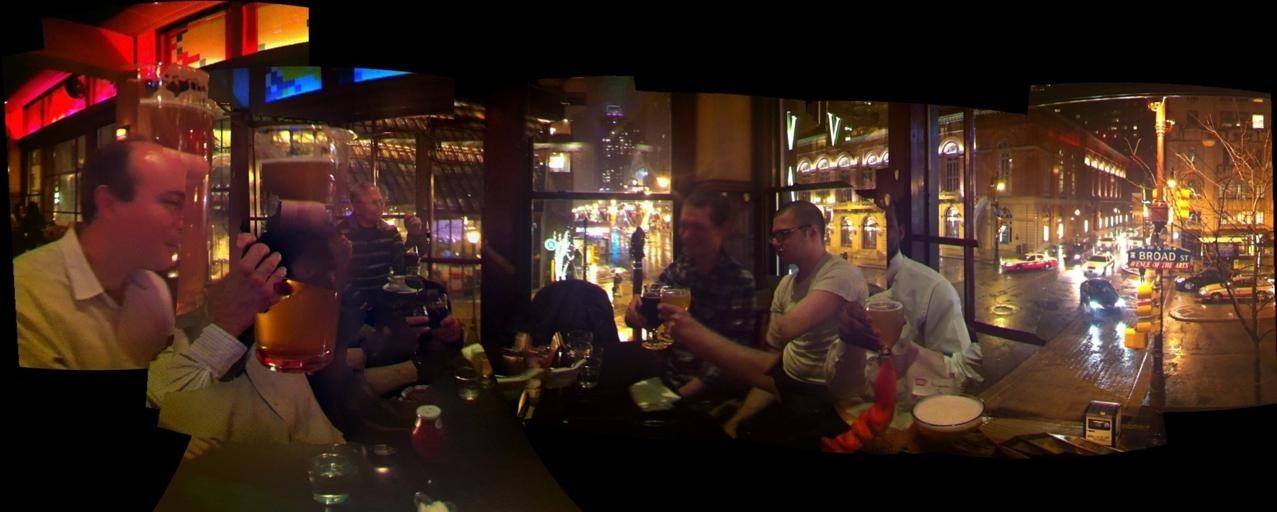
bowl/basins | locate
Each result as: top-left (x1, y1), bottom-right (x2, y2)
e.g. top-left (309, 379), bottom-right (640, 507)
top-left (387, 275), bottom-right (406, 286)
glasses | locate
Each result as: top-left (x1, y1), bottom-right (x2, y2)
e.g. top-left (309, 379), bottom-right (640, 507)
top-left (766, 223), bottom-right (810, 244)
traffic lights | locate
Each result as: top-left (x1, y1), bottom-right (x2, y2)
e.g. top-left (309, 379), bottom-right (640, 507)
top-left (1133, 278), bottom-right (1160, 336)
top-left (1173, 186), bottom-right (1192, 219)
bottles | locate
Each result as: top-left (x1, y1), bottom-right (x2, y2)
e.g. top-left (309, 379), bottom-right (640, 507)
top-left (410, 404), bottom-right (451, 463)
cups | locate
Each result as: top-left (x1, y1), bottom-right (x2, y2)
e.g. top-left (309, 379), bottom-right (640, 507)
top-left (253, 124), bottom-right (355, 374)
top-left (909, 392), bottom-right (986, 462)
top-left (306, 446), bottom-right (352, 505)
top-left (136, 63), bottom-right (215, 316)
top-left (452, 366), bottom-right (482, 408)
top-left (575, 344), bottom-right (604, 389)
top-left (659, 285), bottom-right (691, 339)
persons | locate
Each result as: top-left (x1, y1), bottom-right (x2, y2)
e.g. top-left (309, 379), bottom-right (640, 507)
top-left (5, 135), bottom-right (985, 509)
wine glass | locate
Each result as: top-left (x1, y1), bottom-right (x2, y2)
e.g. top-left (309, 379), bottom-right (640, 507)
top-left (863, 300), bottom-right (906, 362)
top-left (640, 283), bottom-right (668, 351)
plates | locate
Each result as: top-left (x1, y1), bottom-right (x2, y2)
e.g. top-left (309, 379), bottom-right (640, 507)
top-left (382, 282), bottom-right (424, 295)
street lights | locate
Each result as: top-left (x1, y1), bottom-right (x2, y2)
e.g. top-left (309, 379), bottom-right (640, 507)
top-left (1167, 168), bottom-right (1176, 248)
top-left (990, 174), bottom-right (1005, 266)
top-left (464, 227), bottom-right (480, 344)
top-left (1151, 197), bottom-right (1169, 449)
top-left (573, 168), bottom-right (672, 230)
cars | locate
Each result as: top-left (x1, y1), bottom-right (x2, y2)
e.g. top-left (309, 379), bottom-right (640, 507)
top-left (998, 218), bottom-right (1276, 318)
top-left (573, 221), bottom-right (651, 241)
top-left (419, 248), bottom-right (482, 296)
